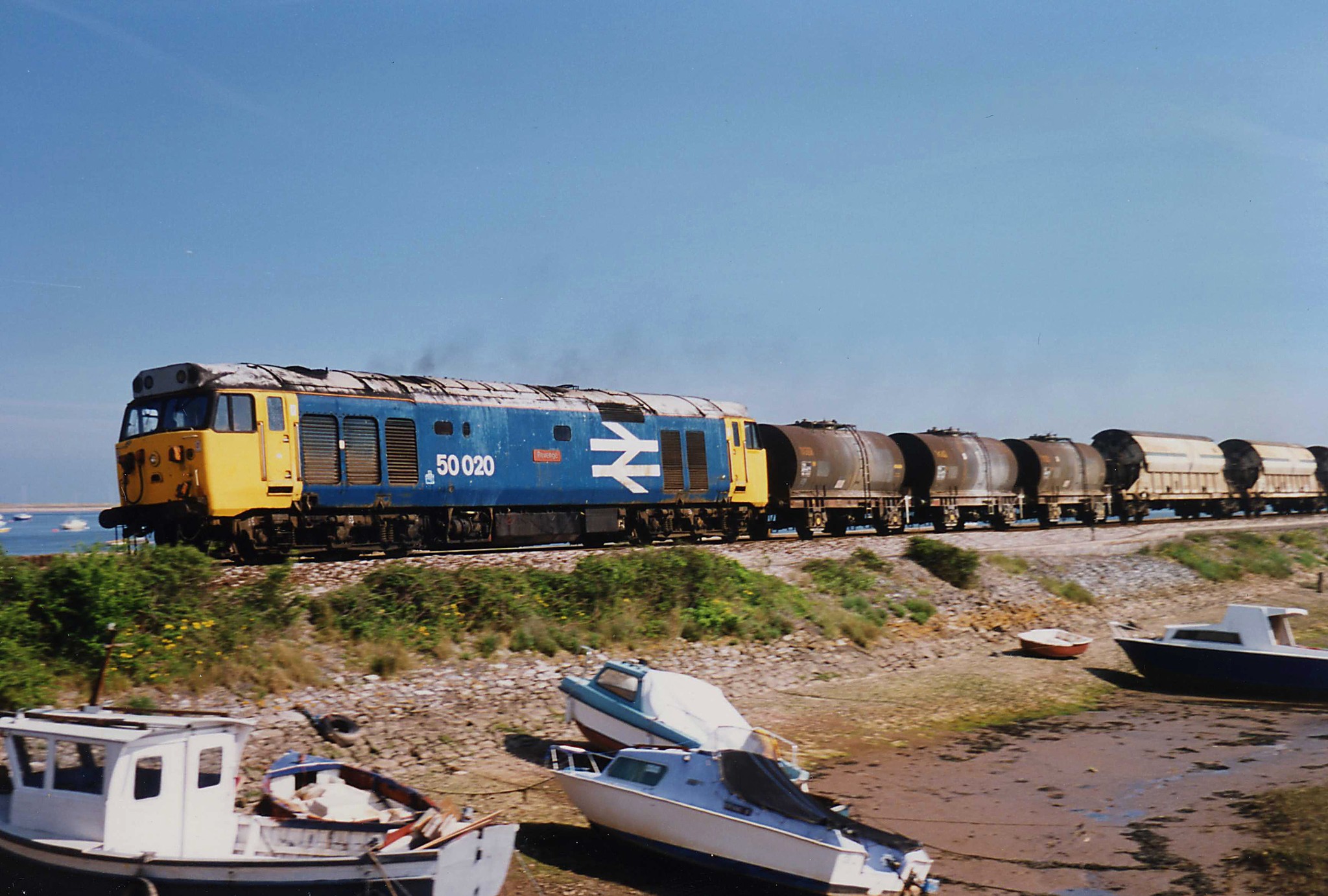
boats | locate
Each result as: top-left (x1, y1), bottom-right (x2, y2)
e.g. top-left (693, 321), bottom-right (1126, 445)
top-left (556, 657), bottom-right (810, 784)
top-left (1108, 605), bottom-right (1328, 691)
top-left (0, 527), bottom-right (10, 533)
top-left (1018, 628), bottom-right (1092, 657)
top-left (546, 725), bottom-right (941, 896)
top-left (105, 537), bottom-right (155, 546)
top-left (14, 514), bottom-right (31, 519)
top-left (0, 521), bottom-right (6, 527)
top-left (0, 515), bottom-right (3, 520)
top-left (60, 520), bottom-right (87, 529)
top-left (0, 644), bottom-right (520, 896)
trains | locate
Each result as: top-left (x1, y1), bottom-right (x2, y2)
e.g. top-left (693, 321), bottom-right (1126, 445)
top-left (99, 362), bottom-right (1328, 559)
